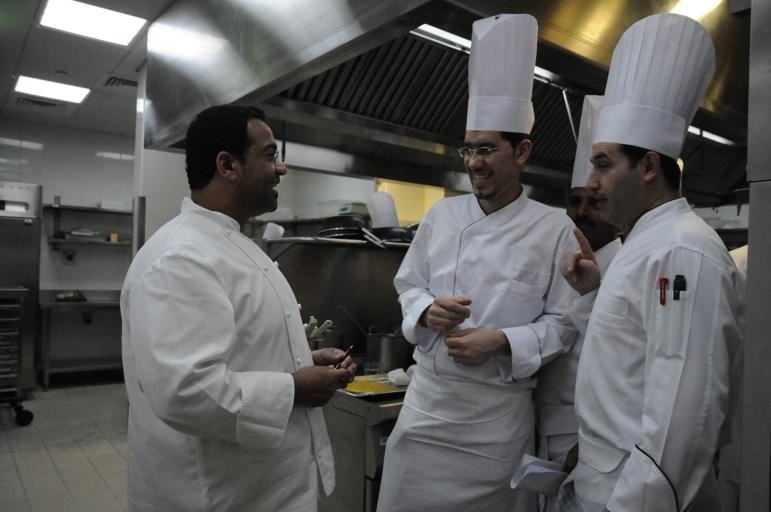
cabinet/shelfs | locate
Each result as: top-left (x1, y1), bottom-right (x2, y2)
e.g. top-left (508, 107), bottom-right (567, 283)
top-left (43, 194), bottom-right (134, 248)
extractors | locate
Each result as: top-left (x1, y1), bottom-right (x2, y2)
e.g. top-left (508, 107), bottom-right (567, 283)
top-left (146, 0), bottom-right (747, 207)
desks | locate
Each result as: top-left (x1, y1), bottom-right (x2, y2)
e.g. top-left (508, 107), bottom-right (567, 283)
top-left (37, 289), bottom-right (122, 390)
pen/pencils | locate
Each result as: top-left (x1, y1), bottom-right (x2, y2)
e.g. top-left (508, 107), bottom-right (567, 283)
top-left (335, 345), bottom-right (354, 369)
top-left (659, 275), bottom-right (686, 305)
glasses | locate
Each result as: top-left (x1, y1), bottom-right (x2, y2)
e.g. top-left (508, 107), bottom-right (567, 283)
top-left (458, 143), bottom-right (516, 158)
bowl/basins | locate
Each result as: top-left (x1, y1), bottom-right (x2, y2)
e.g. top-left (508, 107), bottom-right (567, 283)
top-left (56, 291), bottom-right (85, 302)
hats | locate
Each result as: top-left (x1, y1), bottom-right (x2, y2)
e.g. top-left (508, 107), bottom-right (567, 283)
top-left (591, 13), bottom-right (718, 162)
top-left (262, 223), bottom-right (285, 239)
top-left (366, 191), bottom-right (400, 228)
top-left (569, 94), bottom-right (605, 189)
top-left (465, 12), bottom-right (539, 134)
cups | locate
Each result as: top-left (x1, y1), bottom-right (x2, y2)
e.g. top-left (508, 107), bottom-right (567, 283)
top-left (109, 233), bottom-right (119, 244)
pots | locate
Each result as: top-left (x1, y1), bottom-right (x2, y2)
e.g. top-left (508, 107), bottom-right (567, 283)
top-left (364, 323), bottom-right (416, 373)
top-left (323, 214), bottom-right (422, 250)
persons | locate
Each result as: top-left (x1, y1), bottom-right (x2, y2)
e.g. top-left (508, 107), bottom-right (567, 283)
top-left (552, 138), bottom-right (748, 511)
top-left (533, 184), bottom-right (625, 512)
top-left (119, 103), bottom-right (358, 510)
top-left (375, 125), bottom-right (586, 510)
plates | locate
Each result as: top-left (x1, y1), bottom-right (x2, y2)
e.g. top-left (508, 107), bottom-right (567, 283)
top-left (317, 227), bottom-right (361, 242)
